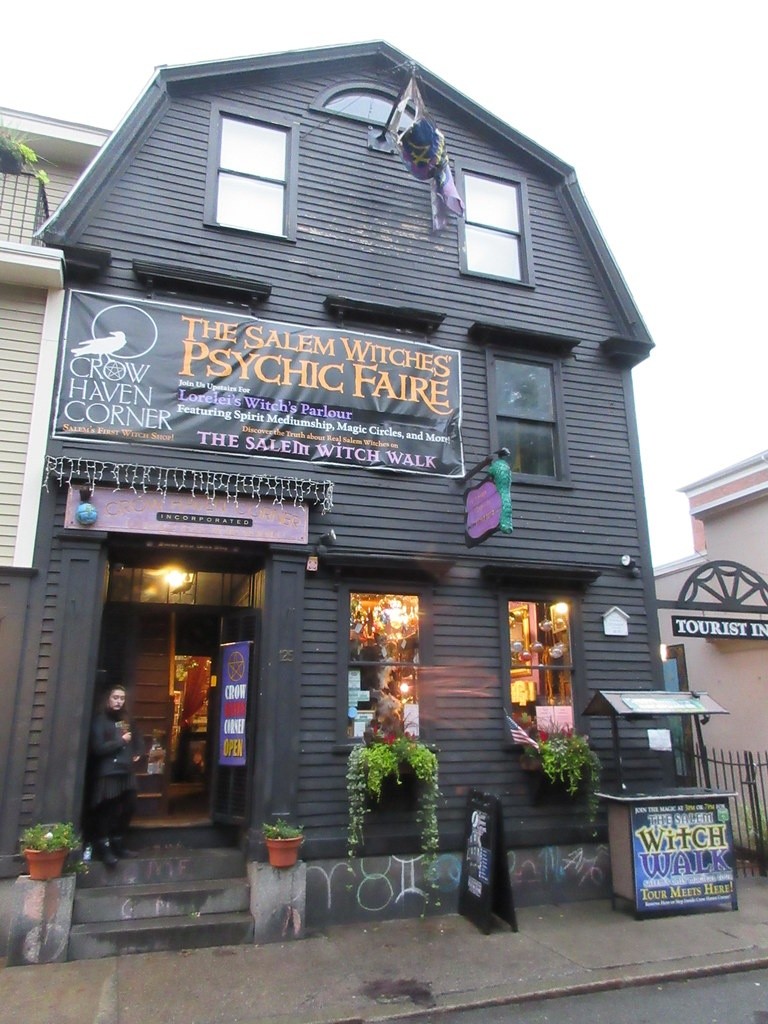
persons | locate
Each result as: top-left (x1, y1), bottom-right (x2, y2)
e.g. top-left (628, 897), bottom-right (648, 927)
top-left (91, 684), bottom-right (145, 864)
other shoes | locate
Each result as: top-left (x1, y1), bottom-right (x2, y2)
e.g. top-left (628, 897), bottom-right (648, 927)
top-left (115, 846), bottom-right (139, 858)
top-left (102, 852), bottom-right (117, 866)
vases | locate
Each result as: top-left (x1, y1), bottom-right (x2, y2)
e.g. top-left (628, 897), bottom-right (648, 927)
top-left (24, 847), bottom-right (68, 881)
top-left (390, 761), bottom-right (413, 773)
top-left (265, 834), bottom-right (303, 868)
top-left (520, 753), bottom-right (541, 770)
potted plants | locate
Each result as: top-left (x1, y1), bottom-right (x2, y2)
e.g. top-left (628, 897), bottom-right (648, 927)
top-left (0, 116), bottom-right (58, 187)
top-left (168, 724), bottom-right (204, 800)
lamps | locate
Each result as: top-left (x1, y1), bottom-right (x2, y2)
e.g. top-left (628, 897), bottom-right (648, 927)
top-left (622, 554), bottom-right (641, 578)
top-left (315, 529), bottom-right (337, 555)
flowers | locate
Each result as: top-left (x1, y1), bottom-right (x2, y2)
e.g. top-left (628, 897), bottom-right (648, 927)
top-left (524, 716), bottom-right (603, 823)
top-left (345, 703), bottom-right (443, 919)
top-left (262, 817), bottom-right (305, 839)
top-left (20, 821), bottom-right (80, 850)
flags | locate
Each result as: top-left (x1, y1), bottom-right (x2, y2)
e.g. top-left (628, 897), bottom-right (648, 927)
top-left (506, 717), bottom-right (540, 755)
top-left (388, 78), bottom-right (465, 232)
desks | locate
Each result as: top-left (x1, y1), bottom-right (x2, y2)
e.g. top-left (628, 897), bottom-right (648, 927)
top-left (594, 787), bottom-right (739, 920)
